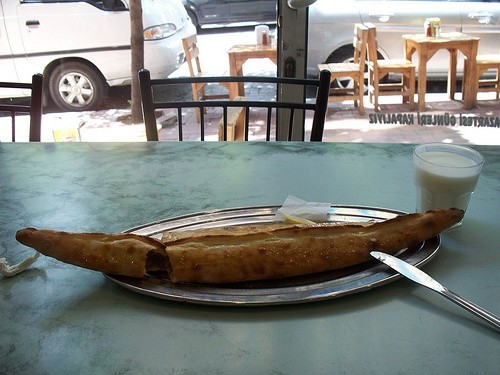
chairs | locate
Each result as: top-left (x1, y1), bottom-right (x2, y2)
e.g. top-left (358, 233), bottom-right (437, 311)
top-left (182, 34), bottom-right (229, 123)
top-left (317, 23), bottom-right (369, 113)
top-left (0, 73), bottom-right (43, 142)
top-left (462, 55), bottom-right (500, 100)
top-left (364, 22), bottom-right (416, 112)
top-left (137, 69), bottom-right (331, 142)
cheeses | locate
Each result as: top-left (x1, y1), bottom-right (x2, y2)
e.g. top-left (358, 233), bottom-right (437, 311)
top-left (285, 215), bottom-right (316, 224)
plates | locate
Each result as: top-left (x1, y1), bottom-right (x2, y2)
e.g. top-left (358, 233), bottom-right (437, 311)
top-left (104, 203), bottom-right (440, 304)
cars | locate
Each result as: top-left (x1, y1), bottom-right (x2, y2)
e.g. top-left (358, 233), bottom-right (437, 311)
top-left (0, 0), bottom-right (198, 113)
top-left (180, 0), bottom-right (276, 33)
top-left (254, 0), bottom-right (500, 97)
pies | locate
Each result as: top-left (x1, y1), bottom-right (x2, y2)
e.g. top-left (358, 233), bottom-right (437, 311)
top-left (162, 207), bottom-right (465, 283)
top-left (15, 227), bottom-right (170, 277)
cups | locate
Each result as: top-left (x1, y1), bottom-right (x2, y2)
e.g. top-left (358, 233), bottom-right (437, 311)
top-left (412, 143), bottom-right (484, 227)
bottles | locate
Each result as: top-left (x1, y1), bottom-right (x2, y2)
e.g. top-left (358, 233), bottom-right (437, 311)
top-left (262, 31), bottom-right (273, 46)
top-left (426, 22), bottom-right (442, 38)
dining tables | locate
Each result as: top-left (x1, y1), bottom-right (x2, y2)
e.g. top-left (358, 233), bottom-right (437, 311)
top-left (0, 143), bottom-right (500, 375)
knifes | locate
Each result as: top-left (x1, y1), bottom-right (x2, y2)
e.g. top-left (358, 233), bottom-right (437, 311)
top-left (370, 251), bottom-right (500, 333)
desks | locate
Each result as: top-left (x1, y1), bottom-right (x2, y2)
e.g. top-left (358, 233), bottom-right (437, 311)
top-left (403, 32), bottom-right (480, 112)
top-left (227, 45), bottom-right (277, 101)
